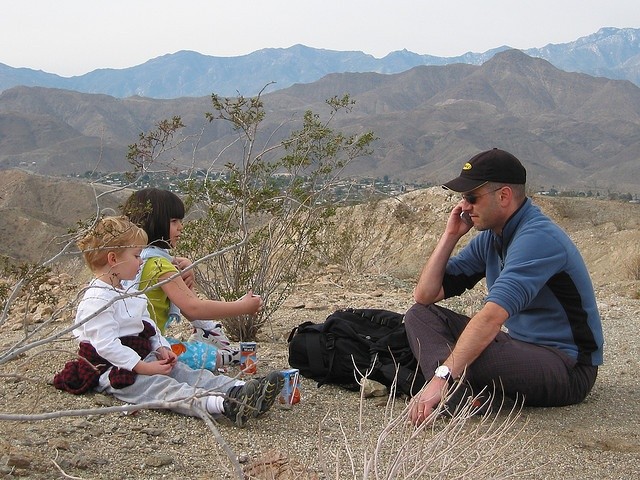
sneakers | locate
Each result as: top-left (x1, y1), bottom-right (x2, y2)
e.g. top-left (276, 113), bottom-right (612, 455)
top-left (432, 379), bottom-right (514, 417)
top-left (239, 371), bottom-right (285, 418)
top-left (217, 347), bottom-right (240, 367)
top-left (221, 379), bottom-right (261, 429)
top-left (188, 324), bottom-right (231, 349)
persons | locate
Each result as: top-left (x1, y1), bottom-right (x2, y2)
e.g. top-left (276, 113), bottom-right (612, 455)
top-left (72, 215), bottom-right (286, 428)
top-left (404, 147), bottom-right (604, 427)
top-left (122, 189), bottom-right (264, 373)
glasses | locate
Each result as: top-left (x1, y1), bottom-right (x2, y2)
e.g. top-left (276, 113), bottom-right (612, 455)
top-left (462, 187), bottom-right (502, 205)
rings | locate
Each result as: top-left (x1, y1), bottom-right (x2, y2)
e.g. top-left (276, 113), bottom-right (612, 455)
top-left (418, 411), bottom-right (423, 413)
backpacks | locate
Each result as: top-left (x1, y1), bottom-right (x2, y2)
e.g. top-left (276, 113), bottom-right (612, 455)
top-left (288, 308), bottom-right (426, 398)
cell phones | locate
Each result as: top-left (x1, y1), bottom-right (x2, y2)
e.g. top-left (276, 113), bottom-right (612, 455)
top-left (459, 210), bottom-right (472, 223)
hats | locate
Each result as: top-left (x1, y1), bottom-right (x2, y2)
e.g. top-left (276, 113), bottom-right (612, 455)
top-left (442, 148), bottom-right (526, 192)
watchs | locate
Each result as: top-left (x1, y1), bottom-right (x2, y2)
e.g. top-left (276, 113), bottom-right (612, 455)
top-left (435, 365), bottom-right (454, 387)
